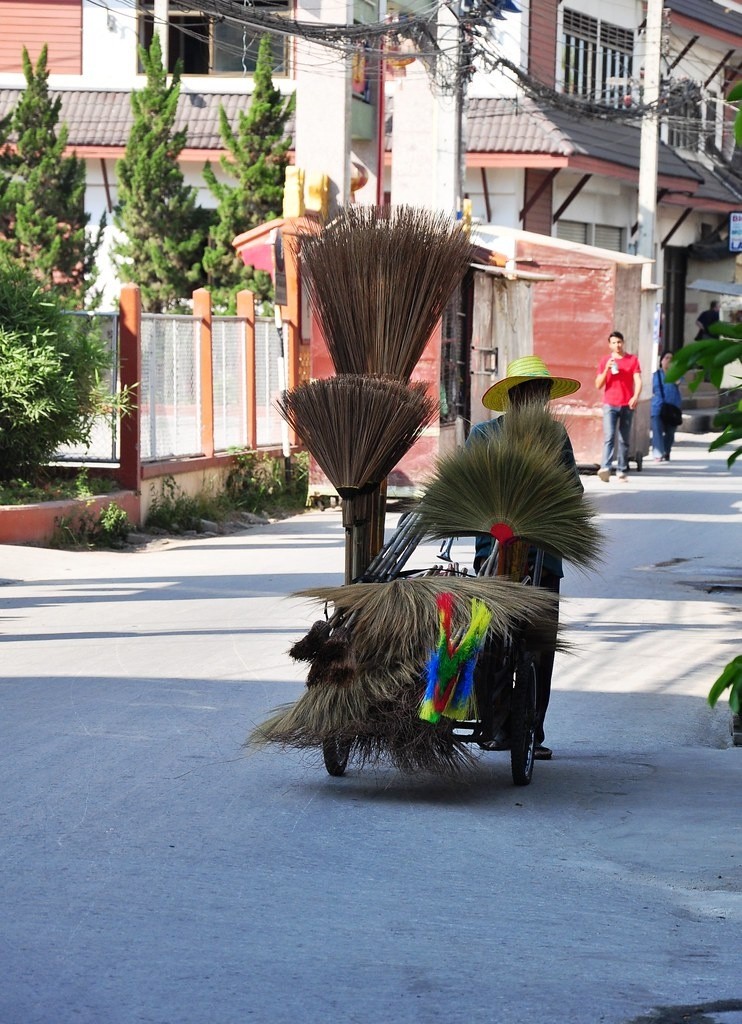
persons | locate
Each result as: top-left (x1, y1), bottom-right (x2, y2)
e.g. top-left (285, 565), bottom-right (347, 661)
top-left (651, 351), bottom-right (686, 462)
top-left (595, 331), bottom-right (642, 482)
top-left (463, 355), bottom-right (584, 758)
top-left (693, 300), bottom-right (720, 383)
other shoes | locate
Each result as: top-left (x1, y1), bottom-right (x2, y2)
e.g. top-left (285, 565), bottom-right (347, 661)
top-left (615, 470), bottom-right (628, 482)
top-left (597, 467), bottom-right (609, 483)
top-left (655, 453), bottom-right (669, 463)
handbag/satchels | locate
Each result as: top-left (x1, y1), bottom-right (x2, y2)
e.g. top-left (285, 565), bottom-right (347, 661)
top-left (659, 402), bottom-right (682, 428)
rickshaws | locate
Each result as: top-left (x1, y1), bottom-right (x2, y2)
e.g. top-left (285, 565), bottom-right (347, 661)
top-left (319, 544), bottom-right (551, 788)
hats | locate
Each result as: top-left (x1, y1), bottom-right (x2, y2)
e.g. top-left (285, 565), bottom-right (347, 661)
top-left (482, 355), bottom-right (581, 412)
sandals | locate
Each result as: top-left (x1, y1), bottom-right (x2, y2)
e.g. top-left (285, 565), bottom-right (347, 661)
top-left (480, 733), bottom-right (509, 751)
top-left (529, 743), bottom-right (552, 759)
